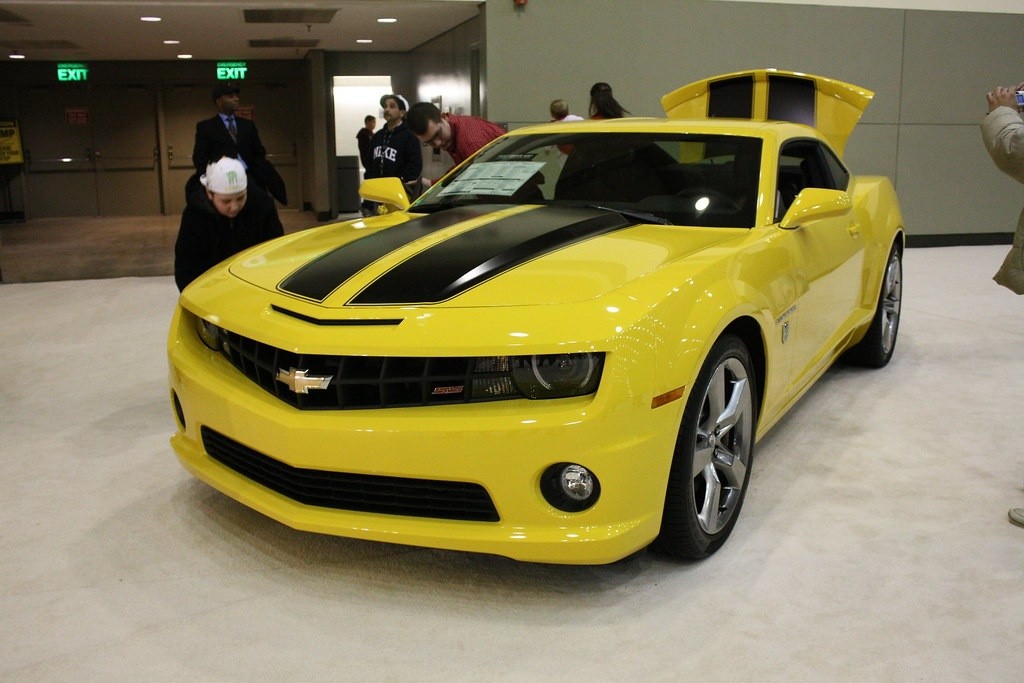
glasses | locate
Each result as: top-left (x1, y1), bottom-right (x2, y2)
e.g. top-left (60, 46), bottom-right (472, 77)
top-left (422, 126), bottom-right (443, 146)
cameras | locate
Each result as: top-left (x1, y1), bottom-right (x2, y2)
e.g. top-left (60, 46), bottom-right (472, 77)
top-left (1014, 91), bottom-right (1024, 108)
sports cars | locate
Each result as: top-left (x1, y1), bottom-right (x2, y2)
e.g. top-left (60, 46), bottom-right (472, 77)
top-left (167, 65), bottom-right (905, 566)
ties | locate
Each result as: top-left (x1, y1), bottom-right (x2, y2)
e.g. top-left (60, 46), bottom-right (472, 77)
top-left (225, 118), bottom-right (238, 144)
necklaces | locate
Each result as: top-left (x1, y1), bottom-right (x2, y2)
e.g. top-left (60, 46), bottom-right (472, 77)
top-left (381, 127), bottom-right (395, 175)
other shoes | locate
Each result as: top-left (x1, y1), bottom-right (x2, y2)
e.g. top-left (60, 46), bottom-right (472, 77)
top-left (1008, 508), bottom-right (1024, 528)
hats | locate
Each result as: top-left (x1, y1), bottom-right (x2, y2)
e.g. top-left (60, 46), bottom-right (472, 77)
top-left (199, 156), bottom-right (248, 194)
top-left (380, 94), bottom-right (409, 112)
top-left (212, 85), bottom-right (240, 101)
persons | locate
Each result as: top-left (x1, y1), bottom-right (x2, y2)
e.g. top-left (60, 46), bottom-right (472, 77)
top-left (544, 83), bottom-right (631, 169)
top-left (980, 78), bottom-right (1024, 531)
top-left (192, 85), bottom-right (268, 193)
top-left (360, 95), bottom-right (423, 219)
top-left (403, 103), bottom-right (546, 198)
top-left (174, 156), bottom-right (283, 293)
top-left (356, 115), bottom-right (376, 171)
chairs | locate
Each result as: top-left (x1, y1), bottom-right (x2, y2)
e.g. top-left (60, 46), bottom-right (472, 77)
top-left (553, 161), bottom-right (806, 228)
